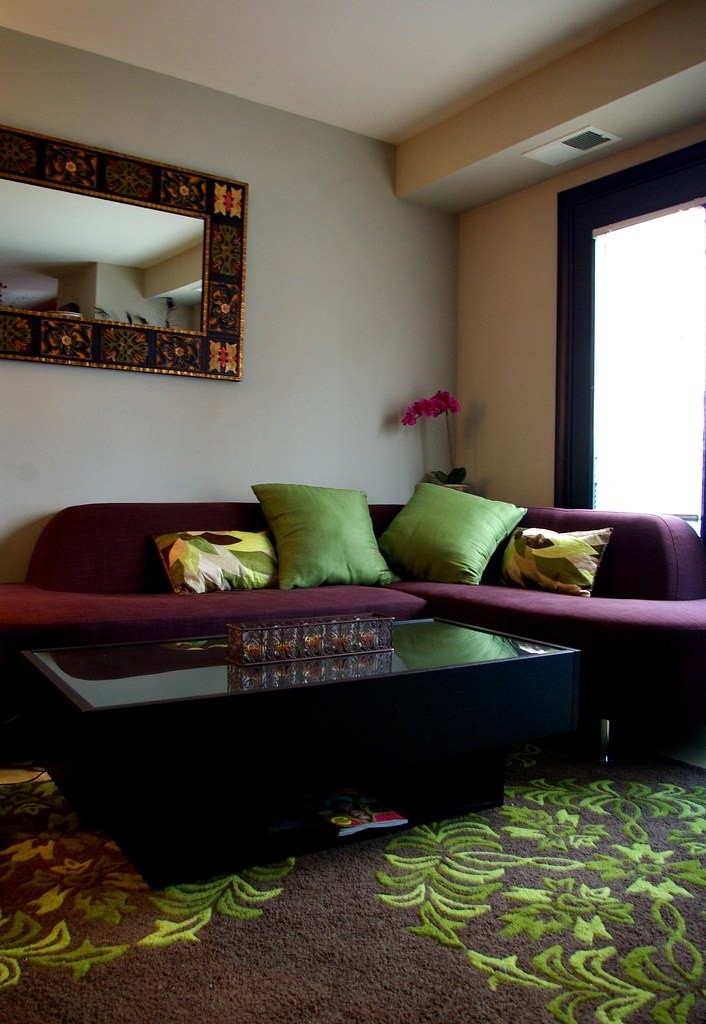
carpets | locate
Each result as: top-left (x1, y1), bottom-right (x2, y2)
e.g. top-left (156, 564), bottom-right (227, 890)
top-left (0, 757), bottom-right (706, 1024)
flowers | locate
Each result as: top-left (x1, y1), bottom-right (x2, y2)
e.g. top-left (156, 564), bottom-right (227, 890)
top-left (401, 390), bottom-right (468, 486)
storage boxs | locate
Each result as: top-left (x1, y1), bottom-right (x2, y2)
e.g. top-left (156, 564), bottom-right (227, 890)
top-left (222, 612), bottom-right (395, 669)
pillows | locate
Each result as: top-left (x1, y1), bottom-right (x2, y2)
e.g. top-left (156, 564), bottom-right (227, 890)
top-left (149, 530), bottom-right (280, 594)
top-left (377, 483), bottom-right (529, 586)
top-left (500, 526), bottom-right (615, 597)
top-left (251, 484), bottom-right (401, 590)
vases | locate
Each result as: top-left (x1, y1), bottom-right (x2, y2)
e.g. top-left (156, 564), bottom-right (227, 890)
top-left (433, 482), bottom-right (475, 496)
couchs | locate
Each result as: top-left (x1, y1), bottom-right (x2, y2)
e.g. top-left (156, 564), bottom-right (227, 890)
top-left (0, 503), bottom-right (426, 769)
top-left (372, 504), bottom-right (706, 765)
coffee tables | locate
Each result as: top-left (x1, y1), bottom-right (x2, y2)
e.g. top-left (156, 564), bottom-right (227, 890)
top-left (18, 617), bottom-right (582, 893)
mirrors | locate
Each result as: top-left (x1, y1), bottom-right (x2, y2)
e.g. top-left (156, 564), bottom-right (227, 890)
top-left (0, 123), bottom-right (250, 381)
